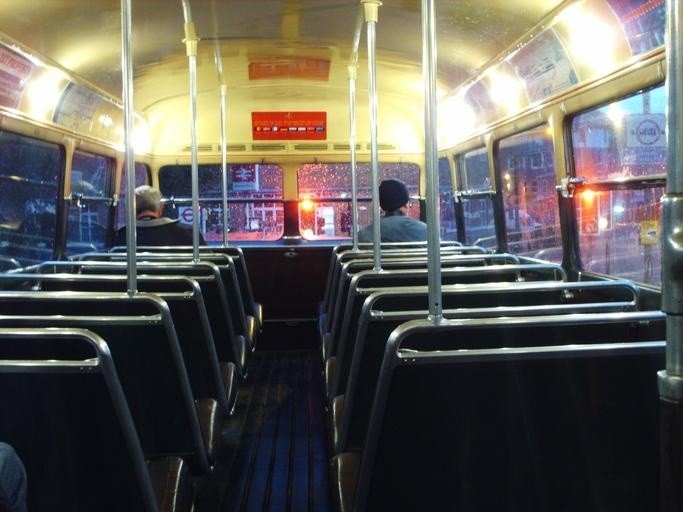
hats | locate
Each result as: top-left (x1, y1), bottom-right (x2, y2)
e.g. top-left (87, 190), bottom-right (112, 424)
top-left (379, 180), bottom-right (409, 211)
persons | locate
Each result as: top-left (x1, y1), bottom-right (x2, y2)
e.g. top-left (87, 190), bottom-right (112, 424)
top-left (351, 178), bottom-right (428, 242)
top-left (116, 184), bottom-right (206, 247)
